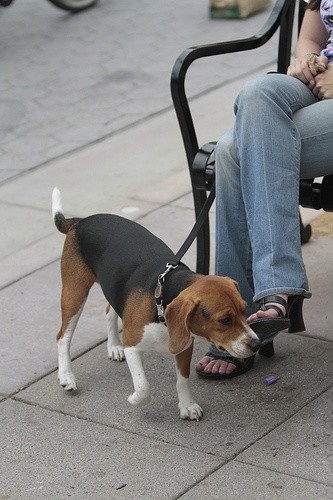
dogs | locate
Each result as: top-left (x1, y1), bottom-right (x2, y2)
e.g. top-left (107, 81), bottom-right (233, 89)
top-left (52, 187), bottom-right (262, 422)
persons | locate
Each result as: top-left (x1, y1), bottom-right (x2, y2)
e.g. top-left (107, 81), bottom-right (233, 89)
top-left (191, 0), bottom-right (333, 380)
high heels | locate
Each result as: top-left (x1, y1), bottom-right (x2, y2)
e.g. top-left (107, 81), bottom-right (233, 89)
top-left (248, 291), bottom-right (307, 337)
top-left (194, 339), bottom-right (275, 379)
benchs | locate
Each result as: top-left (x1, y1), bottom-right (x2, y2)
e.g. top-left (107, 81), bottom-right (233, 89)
top-left (170, 0), bottom-right (333, 276)
